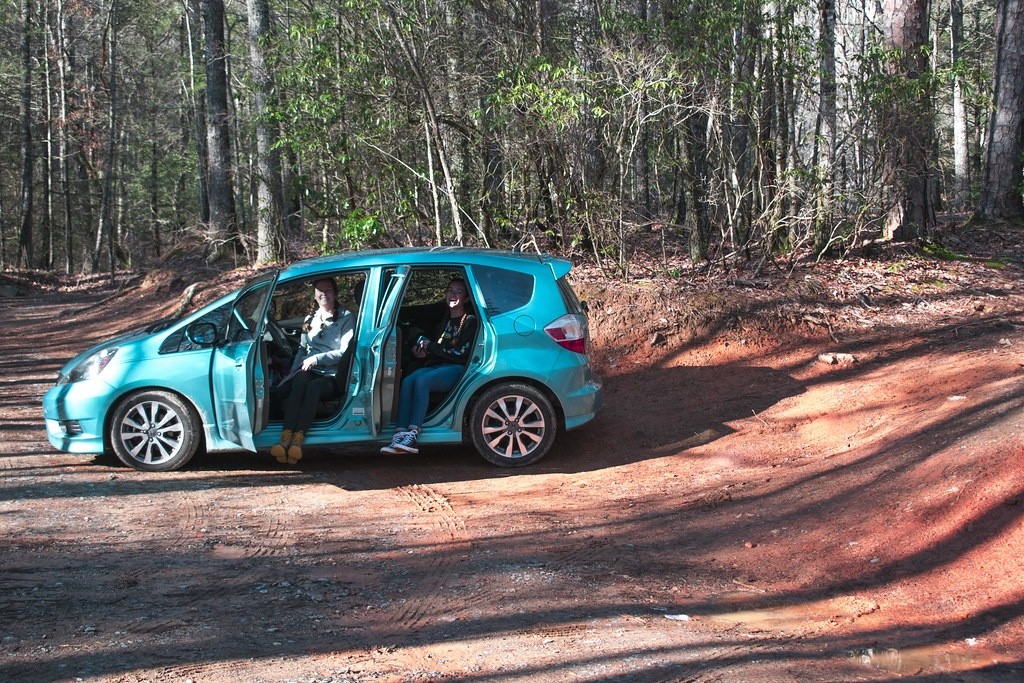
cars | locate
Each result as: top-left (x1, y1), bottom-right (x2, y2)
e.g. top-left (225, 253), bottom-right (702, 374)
top-left (44, 246), bottom-right (602, 470)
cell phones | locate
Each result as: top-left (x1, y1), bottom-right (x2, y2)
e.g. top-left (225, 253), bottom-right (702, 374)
top-left (415, 336), bottom-right (426, 348)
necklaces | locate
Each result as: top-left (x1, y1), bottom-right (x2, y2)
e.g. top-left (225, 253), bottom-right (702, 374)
top-left (449, 313), bottom-right (467, 337)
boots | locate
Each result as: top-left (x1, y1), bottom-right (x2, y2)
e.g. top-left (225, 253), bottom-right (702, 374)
top-left (269, 428), bottom-right (304, 463)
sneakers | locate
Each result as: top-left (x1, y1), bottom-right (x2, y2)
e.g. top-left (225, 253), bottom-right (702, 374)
top-left (380, 427), bottom-right (420, 454)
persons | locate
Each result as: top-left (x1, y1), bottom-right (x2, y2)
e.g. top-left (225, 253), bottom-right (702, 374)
top-left (377, 277), bottom-right (478, 455)
top-left (268, 278), bottom-right (356, 465)
